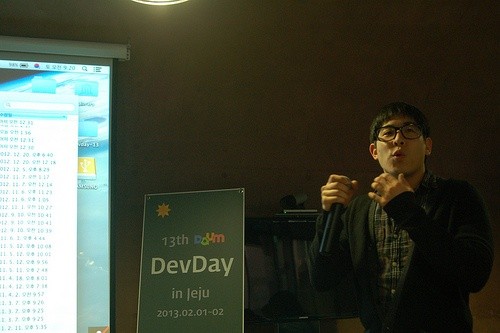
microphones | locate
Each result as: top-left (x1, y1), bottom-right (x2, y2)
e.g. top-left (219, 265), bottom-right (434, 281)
top-left (318, 175), bottom-right (351, 257)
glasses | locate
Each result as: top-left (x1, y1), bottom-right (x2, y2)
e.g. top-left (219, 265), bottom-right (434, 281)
top-left (374, 124), bottom-right (423, 143)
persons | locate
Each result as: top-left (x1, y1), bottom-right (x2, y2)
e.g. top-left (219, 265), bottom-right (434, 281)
top-left (308, 101), bottom-right (495, 333)
